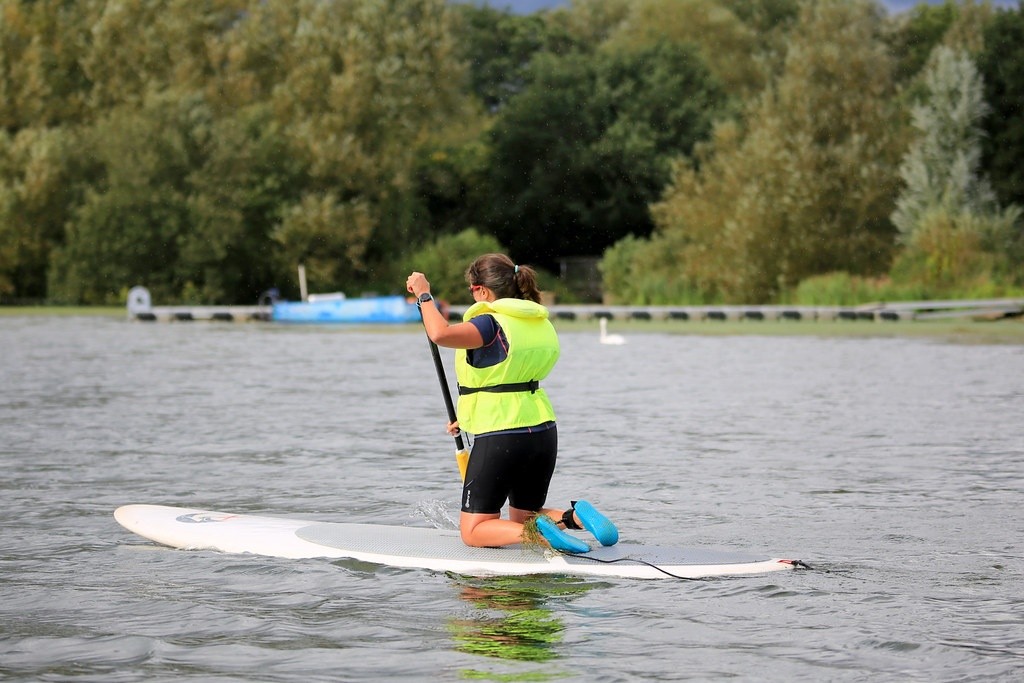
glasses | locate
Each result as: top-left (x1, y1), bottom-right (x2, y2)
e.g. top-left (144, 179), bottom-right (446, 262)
top-left (468, 285), bottom-right (482, 291)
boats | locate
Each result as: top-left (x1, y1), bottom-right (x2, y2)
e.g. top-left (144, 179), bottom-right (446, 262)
top-left (269, 294), bottom-right (440, 322)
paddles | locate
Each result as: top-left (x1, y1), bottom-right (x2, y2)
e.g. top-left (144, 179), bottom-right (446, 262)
top-left (412, 286), bottom-right (470, 483)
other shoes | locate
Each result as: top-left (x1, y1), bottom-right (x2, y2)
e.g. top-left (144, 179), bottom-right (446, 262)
top-left (575, 500), bottom-right (619, 546)
top-left (536, 515), bottom-right (590, 553)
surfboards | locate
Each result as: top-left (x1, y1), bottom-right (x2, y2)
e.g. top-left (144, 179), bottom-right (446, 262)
top-left (112, 504), bottom-right (800, 580)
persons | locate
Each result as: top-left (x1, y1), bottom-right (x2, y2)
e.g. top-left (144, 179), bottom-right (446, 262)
top-left (406, 252), bottom-right (619, 554)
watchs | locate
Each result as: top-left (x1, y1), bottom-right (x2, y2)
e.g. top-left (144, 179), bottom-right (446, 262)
top-left (416, 292), bottom-right (435, 306)
